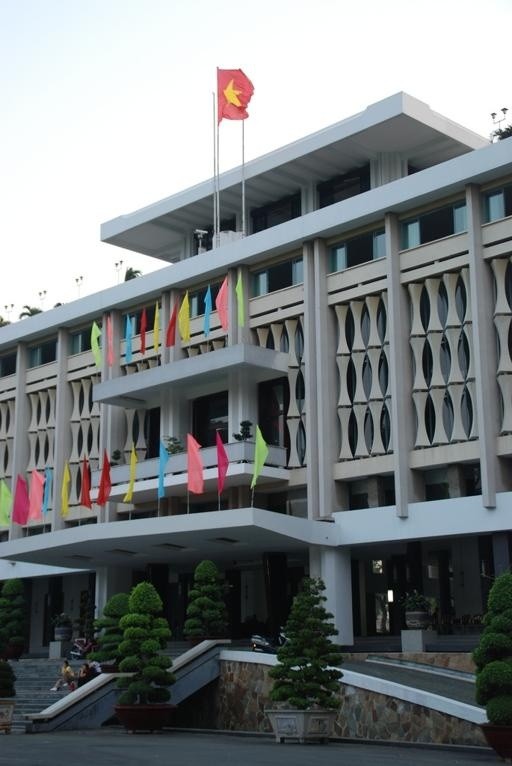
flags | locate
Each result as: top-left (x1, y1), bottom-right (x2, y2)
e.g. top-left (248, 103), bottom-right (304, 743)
top-left (60, 460), bottom-right (70, 518)
top-left (201, 284), bottom-right (213, 337)
top-left (79, 452), bottom-right (92, 504)
top-left (178, 289), bottom-right (193, 343)
top-left (122, 445), bottom-right (139, 504)
top-left (87, 321), bottom-right (103, 370)
top-left (164, 300), bottom-right (178, 348)
top-left (27, 466), bottom-right (42, 522)
top-left (123, 315), bottom-right (134, 364)
top-left (39, 463), bottom-right (49, 518)
top-left (139, 307), bottom-right (148, 354)
top-left (216, 276), bottom-right (230, 334)
top-left (95, 449), bottom-right (113, 506)
top-left (1, 480), bottom-right (10, 526)
top-left (235, 272), bottom-right (246, 328)
top-left (152, 300), bottom-right (161, 352)
top-left (216, 69), bottom-right (256, 125)
top-left (12, 472), bottom-right (29, 526)
top-left (105, 318), bottom-right (114, 367)
top-left (248, 422), bottom-right (270, 491)
top-left (156, 440), bottom-right (169, 499)
top-left (185, 432), bottom-right (206, 494)
top-left (214, 431), bottom-right (230, 494)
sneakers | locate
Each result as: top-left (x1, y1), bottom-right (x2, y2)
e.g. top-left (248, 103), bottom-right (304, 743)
top-left (50, 687), bottom-right (58, 691)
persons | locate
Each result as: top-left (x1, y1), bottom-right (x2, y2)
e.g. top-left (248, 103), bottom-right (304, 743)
top-left (49, 660), bottom-right (74, 692)
top-left (88, 658), bottom-right (102, 677)
top-left (77, 661), bottom-right (90, 687)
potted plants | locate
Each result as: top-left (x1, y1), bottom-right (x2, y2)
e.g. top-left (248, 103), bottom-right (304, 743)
top-left (401, 590), bottom-right (433, 629)
top-left (0, 578), bottom-right (25, 736)
top-left (52, 610), bottom-right (75, 642)
top-left (264, 576), bottom-right (344, 747)
top-left (473, 567), bottom-right (512, 760)
top-left (87, 559), bottom-right (233, 733)
